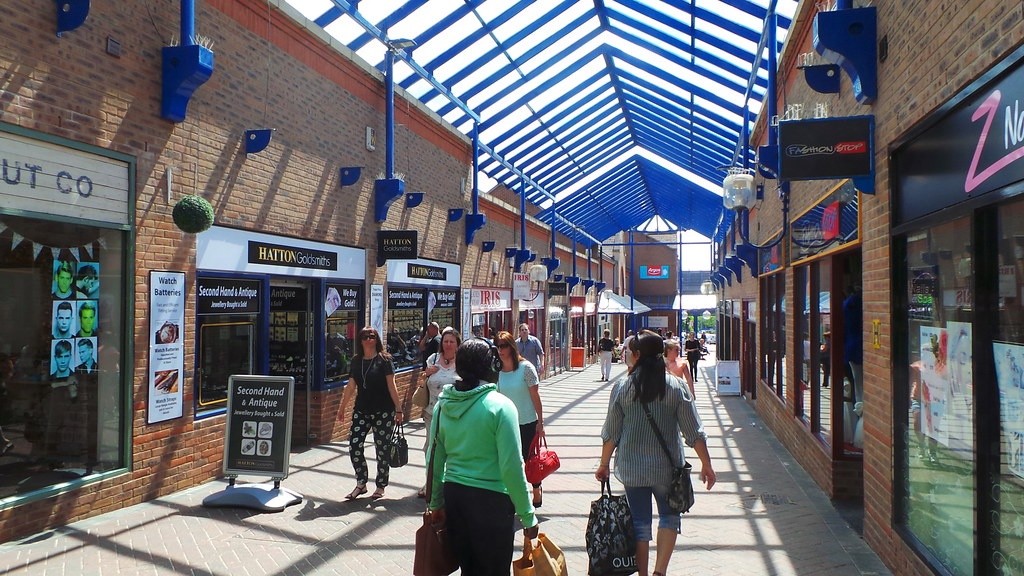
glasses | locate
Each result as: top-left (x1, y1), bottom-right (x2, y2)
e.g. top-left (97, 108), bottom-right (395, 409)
top-left (635, 331), bottom-right (641, 350)
top-left (496, 344), bottom-right (510, 349)
top-left (361, 335), bottom-right (377, 340)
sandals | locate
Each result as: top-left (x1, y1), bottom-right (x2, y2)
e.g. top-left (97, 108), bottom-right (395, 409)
top-left (372, 486), bottom-right (384, 499)
top-left (345, 482), bottom-right (367, 500)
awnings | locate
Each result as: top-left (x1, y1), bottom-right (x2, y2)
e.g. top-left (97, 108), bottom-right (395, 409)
top-left (598, 292), bottom-right (652, 314)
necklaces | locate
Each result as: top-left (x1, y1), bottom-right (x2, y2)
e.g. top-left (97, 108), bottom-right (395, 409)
top-left (443, 353), bottom-right (455, 364)
top-left (362, 354), bottom-right (375, 389)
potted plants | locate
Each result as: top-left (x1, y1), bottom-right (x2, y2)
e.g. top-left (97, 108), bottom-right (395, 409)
top-left (612, 348), bottom-right (621, 363)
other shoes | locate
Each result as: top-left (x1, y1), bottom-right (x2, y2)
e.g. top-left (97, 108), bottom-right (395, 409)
top-left (419, 486), bottom-right (425, 496)
top-left (532, 487), bottom-right (542, 508)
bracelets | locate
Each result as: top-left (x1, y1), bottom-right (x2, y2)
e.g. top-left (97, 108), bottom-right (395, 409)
top-left (396, 412), bottom-right (403, 413)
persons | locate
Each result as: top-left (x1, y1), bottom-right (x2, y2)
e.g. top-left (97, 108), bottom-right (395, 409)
top-left (658, 328), bottom-right (710, 400)
top-left (426, 338), bottom-right (539, 576)
top-left (772, 331), bottom-right (777, 374)
top-left (338, 326), bottom-right (403, 500)
top-left (598, 330), bottom-right (635, 381)
top-left (420, 322), bottom-right (441, 371)
top-left (595, 331), bottom-right (716, 576)
top-left (516, 324), bottom-right (544, 378)
top-left (388, 327), bottom-right (422, 366)
top-left (50, 263), bottom-right (98, 377)
top-left (910, 345), bottom-right (954, 462)
top-left (418, 329), bottom-right (461, 497)
top-left (804, 332), bottom-right (830, 388)
top-left (494, 331), bottom-right (545, 508)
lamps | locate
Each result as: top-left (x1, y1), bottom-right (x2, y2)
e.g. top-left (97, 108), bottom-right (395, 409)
top-left (597, 290), bottom-right (613, 310)
top-left (521, 265), bottom-right (547, 302)
top-left (723, 166), bottom-right (784, 249)
top-left (388, 38), bottom-right (418, 54)
top-left (682, 311), bottom-right (688, 319)
top-left (700, 274), bottom-right (714, 296)
top-left (703, 309), bottom-right (711, 319)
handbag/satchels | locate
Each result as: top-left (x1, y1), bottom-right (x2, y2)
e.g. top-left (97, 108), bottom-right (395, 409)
top-left (512, 527), bottom-right (568, 576)
top-left (388, 420), bottom-right (408, 468)
top-left (666, 464), bottom-right (693, 512)
top-left (586, 476), bottom-right (636, 576)
top-left (412, 352), bottom-right (437, 407)
top-left (414, 509), bottom-right (459, 576)
top-left (525, 429), bottom-right (559, 485)
